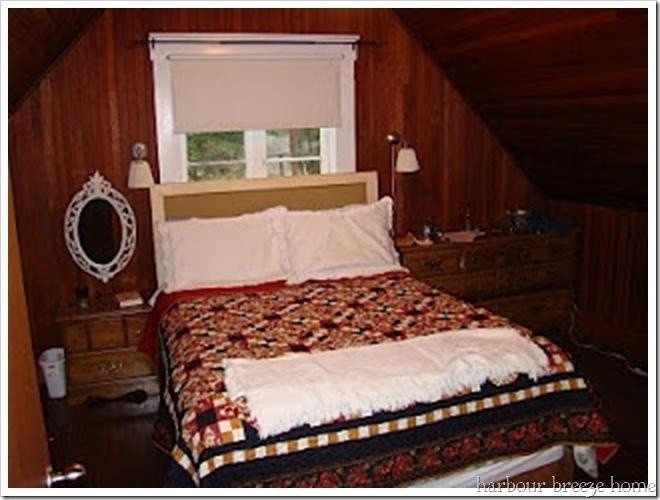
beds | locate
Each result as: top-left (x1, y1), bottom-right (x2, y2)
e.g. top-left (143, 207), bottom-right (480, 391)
top-left (138, 170), bottom-right (622, 488)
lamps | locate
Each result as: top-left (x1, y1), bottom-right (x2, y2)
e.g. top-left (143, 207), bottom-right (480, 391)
top-left (386, 130), bottom-right (422, 194)
top-left (127, 142), bottom-right (154, 190)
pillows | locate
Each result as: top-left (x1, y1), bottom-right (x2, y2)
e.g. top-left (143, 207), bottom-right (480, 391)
top-left (154, 204), bottom-right (289, 294)
top-left (283, 195), bottom-right (410, 286)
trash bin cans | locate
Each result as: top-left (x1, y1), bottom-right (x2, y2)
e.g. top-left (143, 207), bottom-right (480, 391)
top-left (38, 347), bottom-right (67, 399)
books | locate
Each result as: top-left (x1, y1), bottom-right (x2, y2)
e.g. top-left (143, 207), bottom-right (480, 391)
top-left (113, 289), bottom-right (145, 309)
top-left (393, 230), bottom-right (434, 248)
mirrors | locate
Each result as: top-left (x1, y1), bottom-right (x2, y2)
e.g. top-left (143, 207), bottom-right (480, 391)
top-left (63, 171), bottom-right (138, 286)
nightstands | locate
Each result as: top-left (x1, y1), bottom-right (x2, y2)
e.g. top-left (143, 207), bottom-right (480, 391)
top-left (55, 296), bottom-right (159, 407)
top-left (397, 222), bottom-right (582, 336)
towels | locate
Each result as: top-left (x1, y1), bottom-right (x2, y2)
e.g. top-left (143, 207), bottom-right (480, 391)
top-left (221, 327), bottom-right (558, 443)
